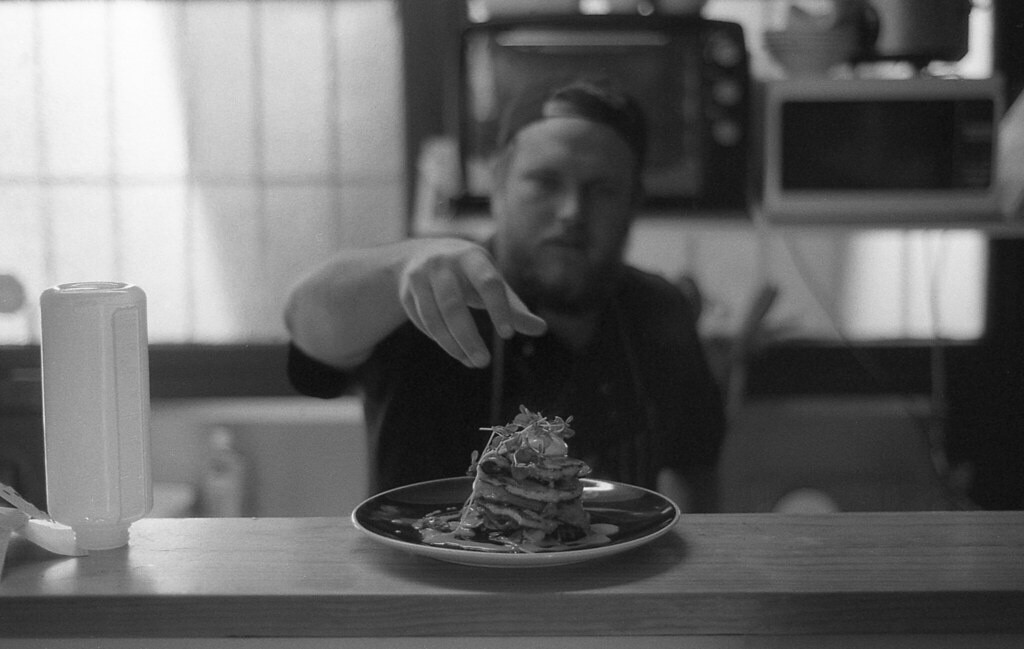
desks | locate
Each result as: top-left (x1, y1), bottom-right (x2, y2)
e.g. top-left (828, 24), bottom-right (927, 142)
top-left (1, 509), bottom-right (1024, 637)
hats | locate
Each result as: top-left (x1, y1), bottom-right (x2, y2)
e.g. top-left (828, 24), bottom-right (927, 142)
top-left (499, 75), bottom-right (649, 180)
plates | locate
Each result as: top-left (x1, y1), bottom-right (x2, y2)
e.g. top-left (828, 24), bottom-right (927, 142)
top-left (350, 475), bottom-right (681, 571)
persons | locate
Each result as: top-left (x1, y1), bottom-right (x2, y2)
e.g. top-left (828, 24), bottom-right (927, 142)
top-left (283, 75), bottom-right (730, 517)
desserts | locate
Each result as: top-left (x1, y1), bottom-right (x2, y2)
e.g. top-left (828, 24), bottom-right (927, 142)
top-left (464, 415), bottom-right (592, 539)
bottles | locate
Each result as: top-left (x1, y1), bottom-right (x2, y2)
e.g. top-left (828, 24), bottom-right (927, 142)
top-left (36, 281), bottom-right (154, 552)
